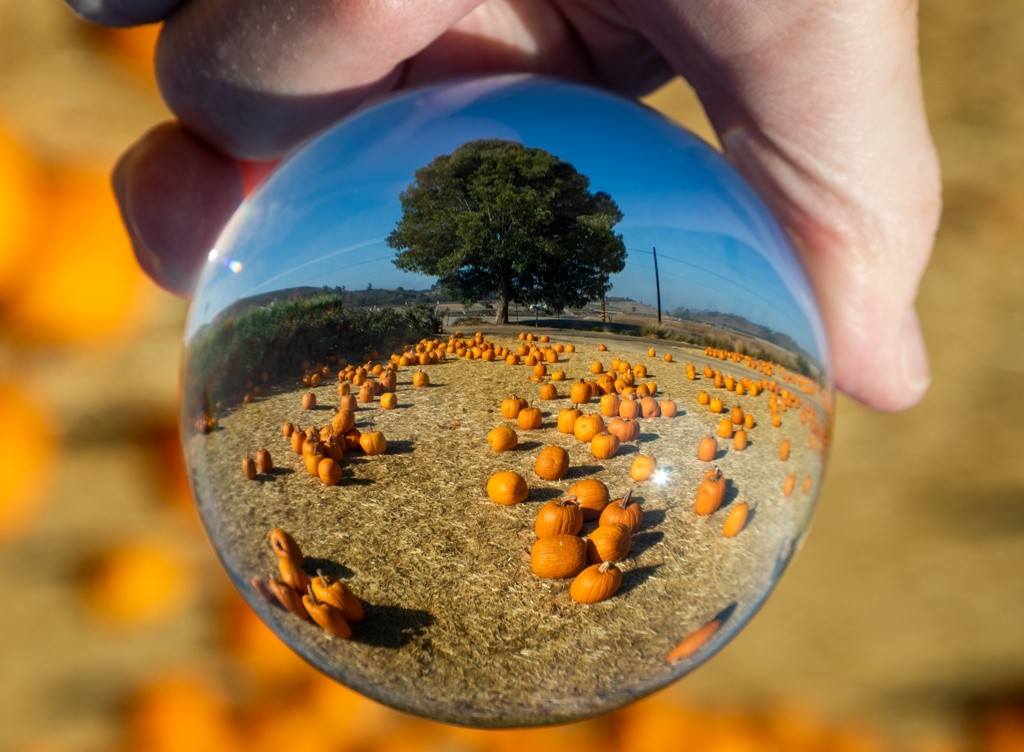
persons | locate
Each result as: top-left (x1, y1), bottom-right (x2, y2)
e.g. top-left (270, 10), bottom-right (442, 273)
top-left (65, 0), bottom-right (944, 411)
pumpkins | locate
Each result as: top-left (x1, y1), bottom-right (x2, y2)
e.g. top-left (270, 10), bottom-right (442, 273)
top-left (187, 330), bottom-right (830, 662)
